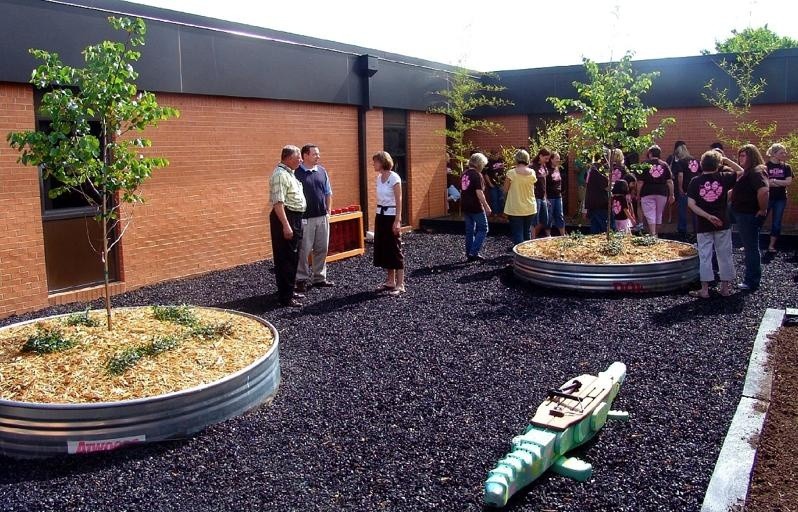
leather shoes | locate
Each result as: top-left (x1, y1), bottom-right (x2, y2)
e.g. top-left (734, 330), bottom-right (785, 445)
top-left (297, 281), bottom-right (307, 291)
top-left (280, 298), bottom-right (302, 307)
top-left (468, 253), bottom-right (484, 260)
top-left (313, 280), bottom-right (335, 287)
top-left (293, 291), bottom-right (305, 299)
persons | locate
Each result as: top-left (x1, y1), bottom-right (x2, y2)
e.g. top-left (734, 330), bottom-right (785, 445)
top-left (291, 143), bottom-right (336, 292)
top-left (686, 150), bottom-right (746, 299)
top-left (266, 144), bottom-right (308, 308)
top-left (372, 151), bottom-right (406, 297)
top-left (729, 144), bottom-right (771, 292)
top-left (446, 138), bottom-right (794, 263)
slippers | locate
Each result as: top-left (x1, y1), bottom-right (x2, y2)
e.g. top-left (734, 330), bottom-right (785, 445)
top-left (376, 284), bottom-right (395, 291)
top-left (388, 288), bottom-right (407, 297)
top-left (768, 248), bottom-right (776, 252)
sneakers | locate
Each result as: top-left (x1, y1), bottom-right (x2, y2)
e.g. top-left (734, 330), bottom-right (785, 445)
top-left (735, 282), bottom-right (753, 289)
top-left (689, 290), bottom-right (709, 298)
top-left (717, 288), bottom-right (730, 296)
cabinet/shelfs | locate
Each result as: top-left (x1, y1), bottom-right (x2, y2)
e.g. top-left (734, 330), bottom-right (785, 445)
top-left (303, 205), bottom-right (365, 266)
